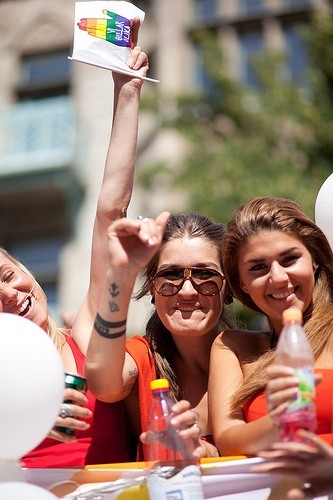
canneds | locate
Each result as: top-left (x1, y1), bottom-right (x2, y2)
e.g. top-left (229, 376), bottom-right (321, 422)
top-left (53, 372), bottom-right (88, 435)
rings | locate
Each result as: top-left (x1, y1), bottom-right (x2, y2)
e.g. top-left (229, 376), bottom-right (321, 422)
top-left (58, 403), bottom-right (72, 417)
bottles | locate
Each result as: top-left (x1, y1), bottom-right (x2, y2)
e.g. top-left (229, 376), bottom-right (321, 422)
top-left (144, 378), bottom-right (202, 500)
top-left (276, 308), bottom-right (316, 444)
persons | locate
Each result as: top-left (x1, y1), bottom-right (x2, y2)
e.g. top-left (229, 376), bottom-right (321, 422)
top-left (0, 17), bottom-right (148, 472)
top-left (208, 196), bottom-right (333, 457)
top-left (85, 211), bottom-right (248, 462)
top-left (250, 429), bottom-right (333, 500)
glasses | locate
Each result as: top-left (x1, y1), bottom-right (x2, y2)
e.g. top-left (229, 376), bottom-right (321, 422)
top-left (150, 268), bottom-right (226, 296)
